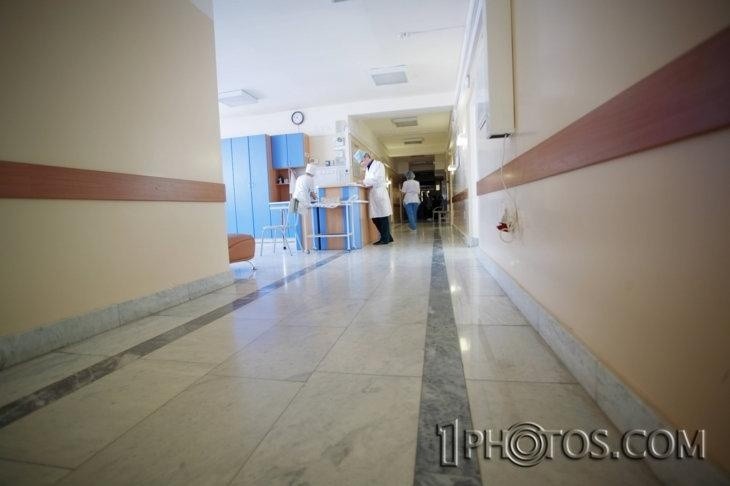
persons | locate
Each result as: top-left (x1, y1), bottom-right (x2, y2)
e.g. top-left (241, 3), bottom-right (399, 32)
top-left (288, 164), bottom-right (318, 251)
top-left (400, 170), bottom-right (421, 231)
top-left (353, 150), bottom-right (394, 245)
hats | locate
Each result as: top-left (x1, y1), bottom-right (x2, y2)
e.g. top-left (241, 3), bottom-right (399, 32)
top-left (353, 150), bottom-right (366, 162)
top-left (306, 164), bottom-right (316, 175)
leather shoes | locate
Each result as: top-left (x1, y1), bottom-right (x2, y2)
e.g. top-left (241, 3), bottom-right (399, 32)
top-left (390, 238), bottom-right (393, 241)
top-left (373, 240), bottom-right (386, 244)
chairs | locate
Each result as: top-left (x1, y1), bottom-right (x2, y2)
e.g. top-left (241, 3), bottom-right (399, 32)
top-left (258, 200), bottom-right (305, 258)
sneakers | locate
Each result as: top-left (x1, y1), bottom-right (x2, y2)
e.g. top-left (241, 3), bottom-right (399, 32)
top-left (407, 225), bottom-right (416, 231)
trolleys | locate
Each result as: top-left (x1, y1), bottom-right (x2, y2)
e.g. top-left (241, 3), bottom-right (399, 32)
top-left (303, 194), bottom-right (358, 253)
top-left (393, 185), bottom-right (405, 228)
